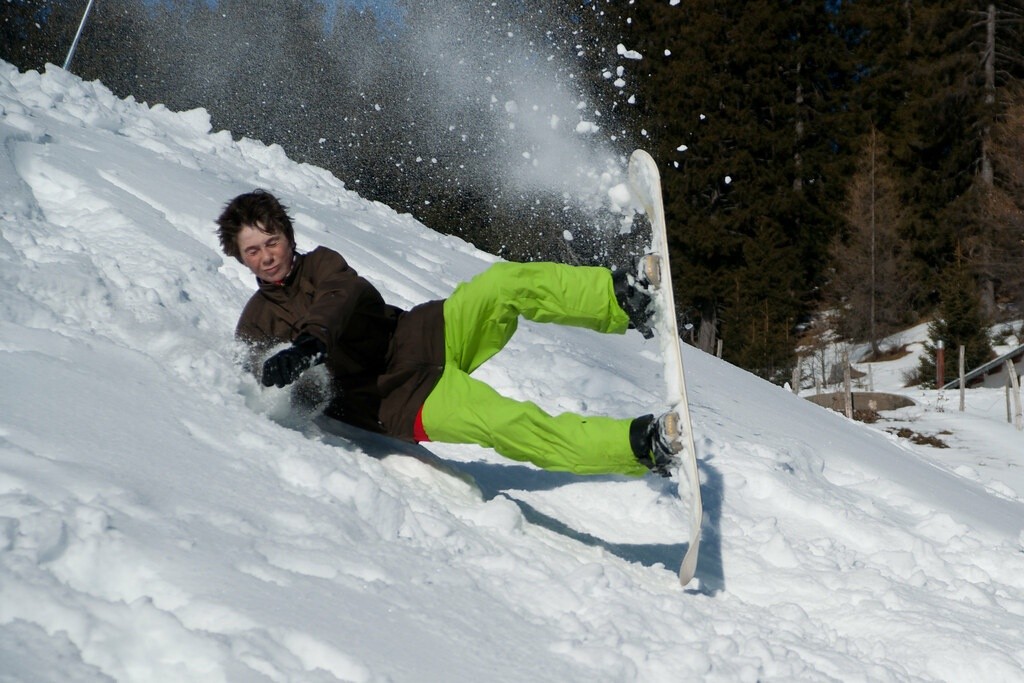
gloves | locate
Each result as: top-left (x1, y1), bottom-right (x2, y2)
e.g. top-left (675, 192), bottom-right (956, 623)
top-left (262, 334), bottom-right (336, 416)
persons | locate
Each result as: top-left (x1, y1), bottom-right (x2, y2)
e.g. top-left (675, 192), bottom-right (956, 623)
top-left (214, 189), bottom-right (682, 481)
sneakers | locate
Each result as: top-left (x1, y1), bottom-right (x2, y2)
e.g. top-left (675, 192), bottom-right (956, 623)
top-left (642, 410), bottom-right (684, 473)
top-left (624, 254), bottom-right (661, 331)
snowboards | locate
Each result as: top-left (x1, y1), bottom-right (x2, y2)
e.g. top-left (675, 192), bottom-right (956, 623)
top-left (623, 146), bottom-right (707, 587)
